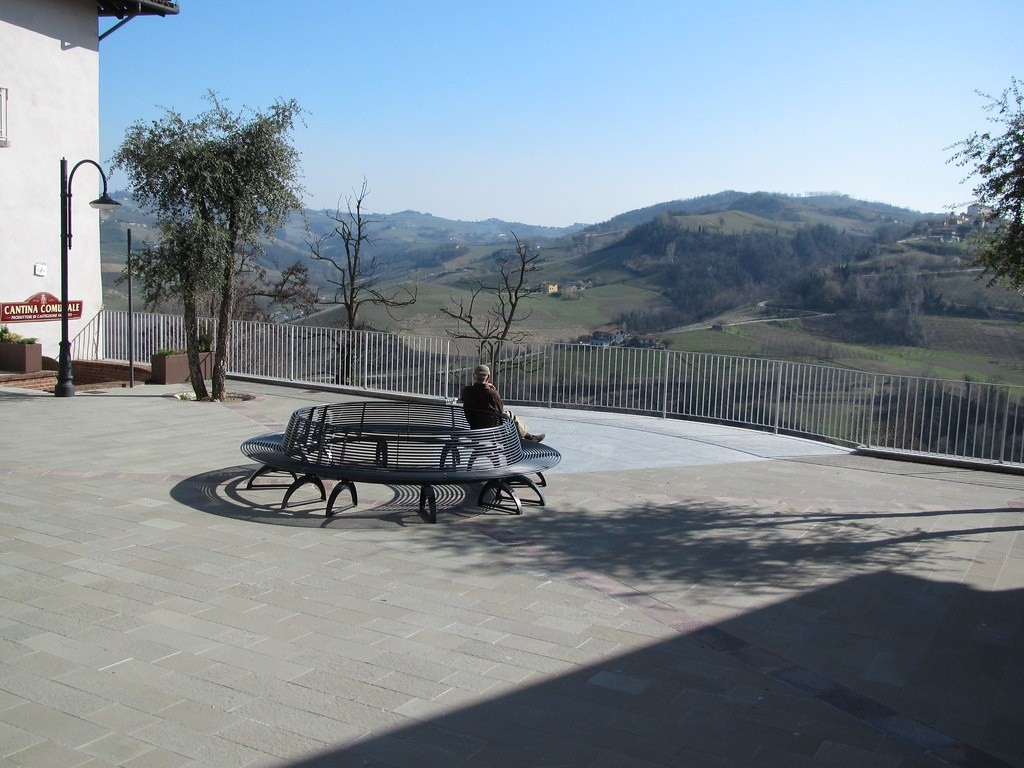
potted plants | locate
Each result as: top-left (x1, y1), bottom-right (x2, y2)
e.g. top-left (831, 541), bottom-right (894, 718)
top-left (153, 329), bottom-right (216, 384)
top-left (0, 325), bottom-right (42, 374)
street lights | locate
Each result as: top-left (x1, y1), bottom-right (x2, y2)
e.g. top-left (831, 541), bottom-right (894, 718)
top-left (55, 157), bottom-right (123, 397)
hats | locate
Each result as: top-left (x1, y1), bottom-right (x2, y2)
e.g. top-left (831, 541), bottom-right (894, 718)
top-left (475, 365), bottom-right (490, 376)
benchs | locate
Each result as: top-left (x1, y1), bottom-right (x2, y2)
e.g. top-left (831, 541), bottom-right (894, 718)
top-left (239, 402), bottom-right (561, 523)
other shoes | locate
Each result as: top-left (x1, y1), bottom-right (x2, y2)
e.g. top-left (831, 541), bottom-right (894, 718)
top-left (532, 434), bottom-right (545, 443)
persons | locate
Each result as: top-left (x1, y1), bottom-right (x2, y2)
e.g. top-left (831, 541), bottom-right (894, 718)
top-left (461, 365), bottom-right (545, 443)
